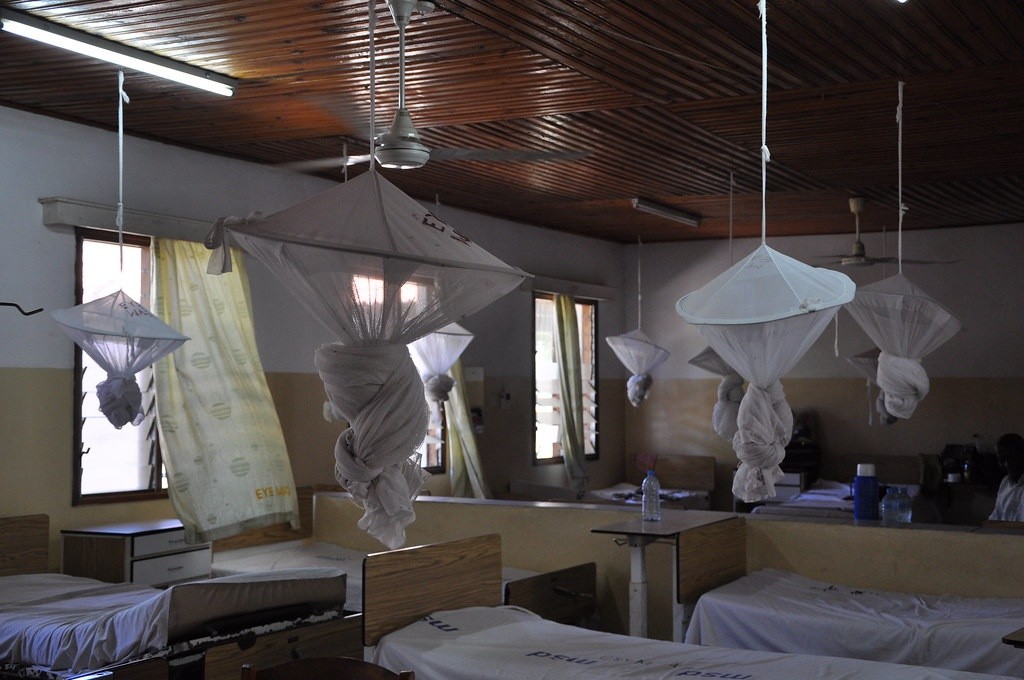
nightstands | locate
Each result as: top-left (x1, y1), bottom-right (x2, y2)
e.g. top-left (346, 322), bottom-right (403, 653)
top-left (759, 472), bottom-right (805, 505)
top-left (58, 519), bottom-right (214, 593)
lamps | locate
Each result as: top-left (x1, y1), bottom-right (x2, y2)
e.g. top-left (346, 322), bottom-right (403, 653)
top-left (630, 199), bottom-right (700, 227)
top-left (1, 7), bottom-right (238, 98)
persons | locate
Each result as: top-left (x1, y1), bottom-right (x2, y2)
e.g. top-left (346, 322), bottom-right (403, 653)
top-left (988, 432), bottom-right (1024, 522)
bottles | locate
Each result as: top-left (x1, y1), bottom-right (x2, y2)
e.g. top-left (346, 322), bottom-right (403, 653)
top-left (641, 470), bottom-right (661, 521)
top-left (853, 463), bottom-right (879, 527)
top-left (881, 486), bottom-right (912, 526)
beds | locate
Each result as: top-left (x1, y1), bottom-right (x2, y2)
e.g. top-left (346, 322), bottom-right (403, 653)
top-left (0, 456), bottom-right (1024, 680)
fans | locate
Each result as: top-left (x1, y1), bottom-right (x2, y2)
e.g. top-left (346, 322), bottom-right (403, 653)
top-left (810, 198), bottom-right (963, 266)
top-left (264, 0), bottom-right (590, 171)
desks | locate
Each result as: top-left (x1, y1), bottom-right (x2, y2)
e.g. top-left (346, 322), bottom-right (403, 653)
top-left (589, 512), bottom-right (747, 641)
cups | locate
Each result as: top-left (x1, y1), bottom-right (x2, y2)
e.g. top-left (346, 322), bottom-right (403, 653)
top-left (947, 473), bottom-right (960, 482)
